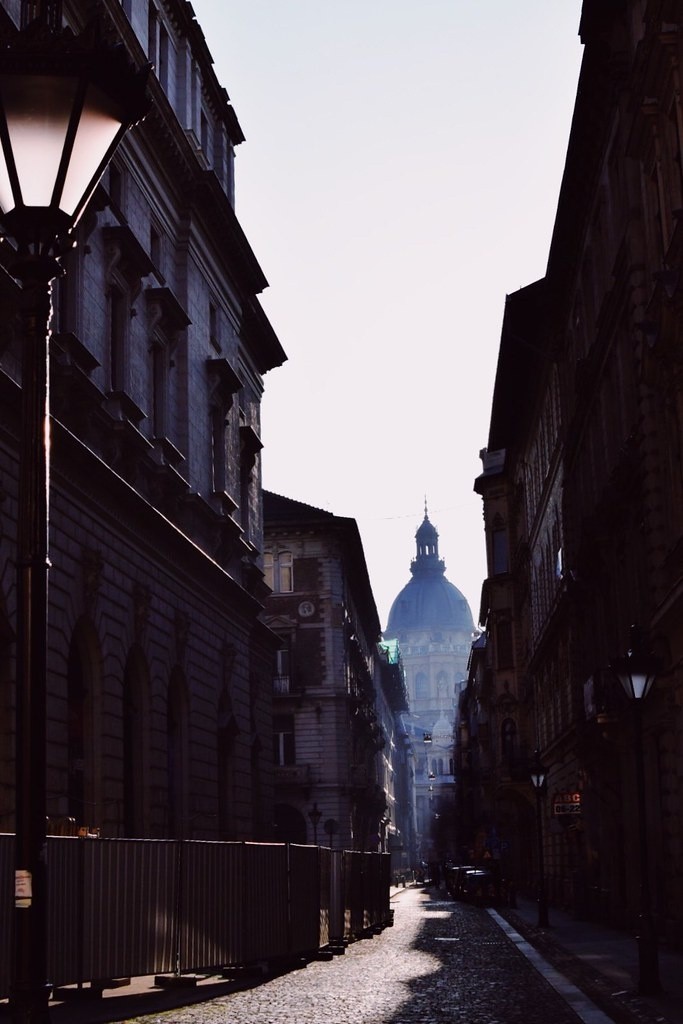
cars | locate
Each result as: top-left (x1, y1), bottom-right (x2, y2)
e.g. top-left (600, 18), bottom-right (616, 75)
top-left (449, 865), bottom-right (506, 904)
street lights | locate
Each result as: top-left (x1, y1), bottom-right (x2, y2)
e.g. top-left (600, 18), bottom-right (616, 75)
top-left (0, 38), bottom-right (156, 1024)
top-left (527, 748), bottom-right (550, 930)
top-left (609, 622), bottom-right (668, 998)
top-left (307, 802), bottom-right (323, 951)
